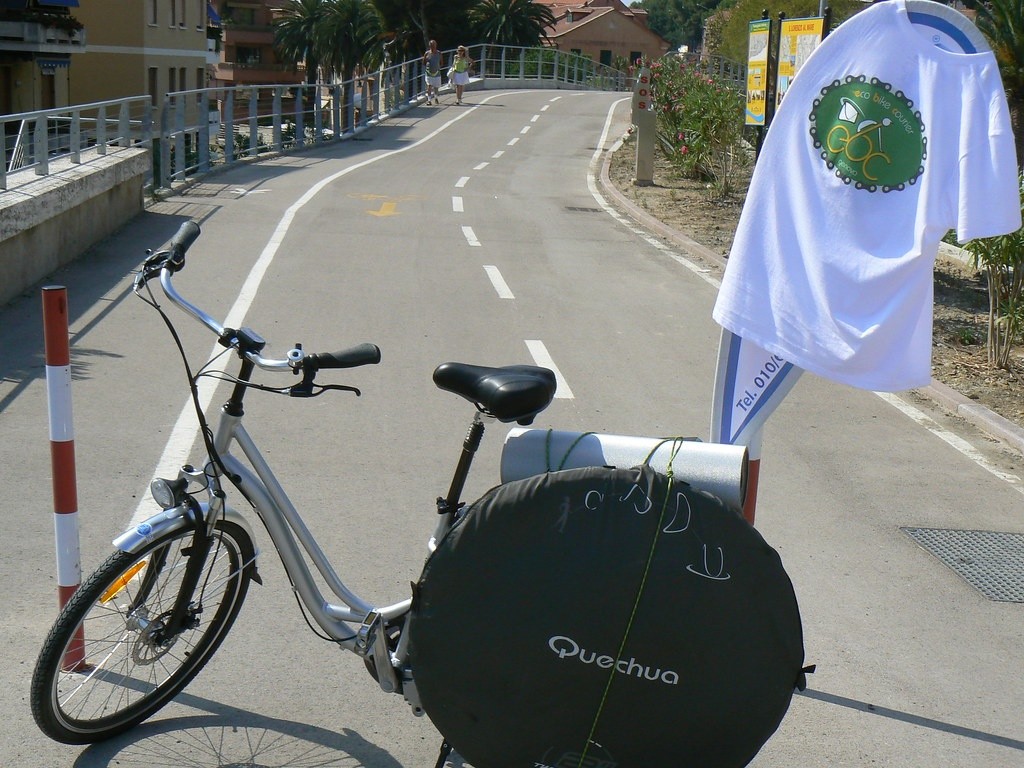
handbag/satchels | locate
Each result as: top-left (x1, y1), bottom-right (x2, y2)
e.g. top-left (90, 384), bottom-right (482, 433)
top-left (447, 66), bottom-right (454, 79)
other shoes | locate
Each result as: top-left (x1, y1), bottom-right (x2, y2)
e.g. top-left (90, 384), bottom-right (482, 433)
top-left (457, 99), bottom-right (462, 103)
top-left (435, 98), bottom-right (438, 106)
top-left (427, 100), bottom-right (431, 105)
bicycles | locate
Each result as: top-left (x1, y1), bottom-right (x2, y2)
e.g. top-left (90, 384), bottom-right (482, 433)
top-left (30, 221), bottom-right (557, 768)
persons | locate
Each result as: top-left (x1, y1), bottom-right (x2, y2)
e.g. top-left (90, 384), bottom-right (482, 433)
top-left (450, 46), bottom-right (475, 104)
top-left (421, 40), bottom-right (444, 106)
